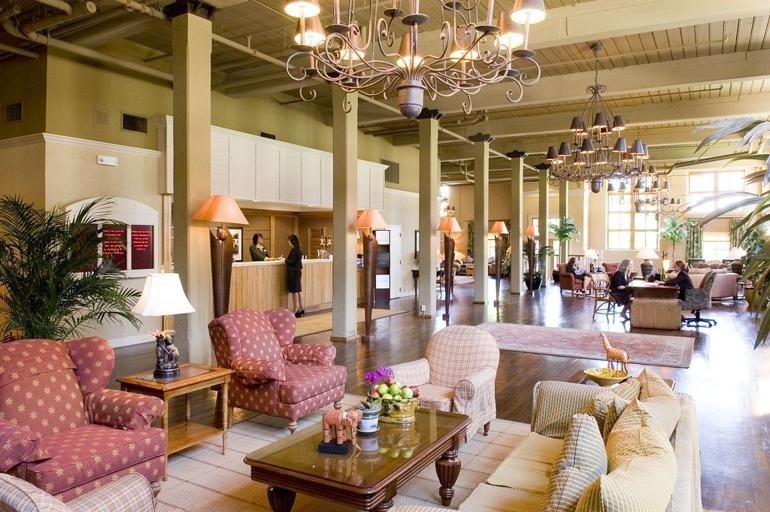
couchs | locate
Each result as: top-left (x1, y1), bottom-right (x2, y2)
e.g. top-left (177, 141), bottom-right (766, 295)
top-left (388, 379), bottom-right (700, 512)
top-left (0, 473), bottom-right (157, 512)
top-left (670, 273), bottom-right (739, 299)
top-left (386, 325), bottom-right (499, 444)
top-left (209, 309), bottom-right (347, 433)
top-left (556, 263), bottom-right (583, 295)
top-left (602, 263), bottom-right (638, 283)
top-left (0, 337), bottom-right (168, 503)
top-left (440, 259), bottom-right (462, 272)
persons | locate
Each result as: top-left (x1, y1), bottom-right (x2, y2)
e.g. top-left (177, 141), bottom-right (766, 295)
top-left (657, 261), bottom-right (694, 323)
top-left (249, 233), bottom-right (276, 260)
top-left (610, 259), bottom-right (633, 320)
top-left (285, 234), bottom-right (305, 317)
top-left (566, 258), bottom-right (590, 295)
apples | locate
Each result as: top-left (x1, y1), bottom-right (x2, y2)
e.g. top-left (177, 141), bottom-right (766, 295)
top-left (371, 384), bottom-right (414, 399)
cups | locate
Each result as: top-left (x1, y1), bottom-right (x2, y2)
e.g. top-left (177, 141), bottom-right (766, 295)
top-left (322, 250), bottom-right (327, 259)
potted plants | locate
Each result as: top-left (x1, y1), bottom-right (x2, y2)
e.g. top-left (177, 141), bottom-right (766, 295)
top-left (549, 216), bottom-right (583, 283)
top-left (727, 217), bottom-right (769, 307)
top-left (522, 242), bottom-right (560, 291)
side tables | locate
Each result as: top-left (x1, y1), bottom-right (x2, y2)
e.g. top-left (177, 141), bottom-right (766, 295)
top-left (115, 362), bottom-right (237, 456)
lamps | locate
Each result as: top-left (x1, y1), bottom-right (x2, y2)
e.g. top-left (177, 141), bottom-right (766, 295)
top-left (584, 249), bottom-right (599, 273)
top-left (435, 216), bottom-right (463, 319)
top-left (545, 52), bottom-right (681, 219)
top-left (351, 208), bottom-right (387, 343)
top-left (634, 247), bottom-right (660, 279)
top-left (130, 272), bottom-right (196, 379)
top-left (488, 221), bottom-right (510, 306)
top-left (283, 0), bottom-right (547, 120)
top-left (192, 194), bottom-right (250, 319)
top-left (522, 225), bottom-right (536, 294)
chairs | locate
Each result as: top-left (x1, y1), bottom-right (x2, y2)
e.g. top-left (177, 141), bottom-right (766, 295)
top-left (591, 278), bottom-right (617, 319)
top-left (683, 271), bottom-right (716, 327)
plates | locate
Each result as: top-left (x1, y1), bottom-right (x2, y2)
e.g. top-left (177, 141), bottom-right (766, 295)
top-left (356, 434), bottom-right (379, 440)
top-left (356, 426), bottom-right (381, 435)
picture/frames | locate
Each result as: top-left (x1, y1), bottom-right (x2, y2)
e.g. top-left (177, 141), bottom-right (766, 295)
top-left (217, 227), bottom-right (244, 262)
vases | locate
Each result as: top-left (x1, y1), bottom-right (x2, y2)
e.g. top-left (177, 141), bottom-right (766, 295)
top-left (357, 411), bottom-right (383, 433)
top-left (354, 434), bottom-right (383, 464)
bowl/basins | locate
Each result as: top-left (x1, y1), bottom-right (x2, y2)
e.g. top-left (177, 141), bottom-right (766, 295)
top-left (584, 368), bottom-right (630, 386)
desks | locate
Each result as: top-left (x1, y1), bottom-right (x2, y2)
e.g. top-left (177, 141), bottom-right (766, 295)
top-left (626, 279), bottom-right (680, 298)
top-left (588, 273), bottom-right (610, 293)
top-left (411, 270), bottom-right (442, 294)
top-left (243, 403), bottom-right (473, 512)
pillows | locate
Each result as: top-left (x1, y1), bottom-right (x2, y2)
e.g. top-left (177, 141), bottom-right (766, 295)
top-left (687, 268), bottom-right (727, 273)
top-left (538, 412), bottom-right (609, 512)
top-left (574, 399), bottom-right (676, 512)
top-left (586, 376), bottom-right (640, 438)
top-left (604, 367), bottom-right (681, 444)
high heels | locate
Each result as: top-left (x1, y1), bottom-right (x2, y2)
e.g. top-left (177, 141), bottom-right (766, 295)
top-left (300, 309), bottom-right (305, 317)
top-left (295, 311), bottom-right (300, 317)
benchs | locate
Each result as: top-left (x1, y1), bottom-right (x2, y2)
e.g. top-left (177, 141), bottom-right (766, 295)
top-left (629, 298), bottom-right (682, 330)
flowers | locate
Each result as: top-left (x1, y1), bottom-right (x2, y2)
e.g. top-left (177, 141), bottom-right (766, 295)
top-left (364, 368), bottom-right (395, 408)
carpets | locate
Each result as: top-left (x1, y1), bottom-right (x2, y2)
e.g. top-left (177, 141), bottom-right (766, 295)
top-left (473, 321), bottom-right (696, 369)
